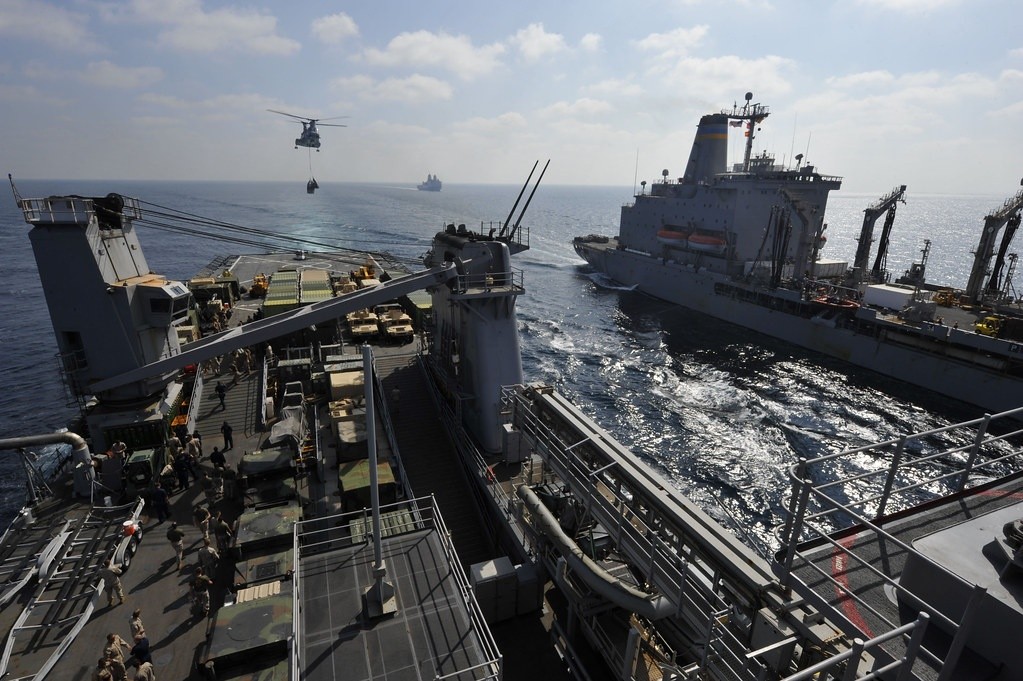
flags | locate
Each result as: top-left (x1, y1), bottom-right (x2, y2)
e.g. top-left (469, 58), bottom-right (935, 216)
top-left (731, 121), bottom-right (743, 127)
top-left (747, 122), bottom-right (752, 128)
top-left (745, 132), bottom-right (750, 136)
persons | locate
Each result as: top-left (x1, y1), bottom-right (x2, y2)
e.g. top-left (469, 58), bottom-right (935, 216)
top-left (211, 511), bottom-right (234, 553)
top-left (166, 521), bottom-right (185, 570)
top-left (221, 420), bottom-right (234, 450)
top-left (99, 558), bottom-right (125, 607)
top-left (193, 504), bottom-right (212, 545)
top-left (189, 565), bottom-right (213, 617)
top-left (198, 538), bottom-right (218, 577)
top-left (92, 632), bottom-right (157, 681)
top-left (110, 437), bottom-right (127, 464)
top-left (152, 422), bottom-right (236, 521)
top-left (391, 383), bottom-right (402, 412)
top-left (127, 607), bottom-right (146, 637)
top-left (214, 381), bottom-right (226, 410)
top-left (239, 308), bottom-right (262, 326)
top-left (265, 343), bottom-right (274, 367)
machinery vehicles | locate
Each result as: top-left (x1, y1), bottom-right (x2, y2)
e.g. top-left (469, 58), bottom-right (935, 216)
top-left (354, 266), bottom-right (414, 344)
top-left (932, 290), bottom-right (960, 306)
top-left (334, 275), bottom-right (379, 341)
top-left (249, 273), bottom-right (268, 298)
top-left (975, 317), bottom-right (1001, 338)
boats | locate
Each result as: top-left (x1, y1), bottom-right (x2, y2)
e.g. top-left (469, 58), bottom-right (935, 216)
top-left (814, 294), bottom-right (858, 308)
top-left (573, 93), bottom-right (1023, 423)
top-left (417, 174), bottom-right (441, 192)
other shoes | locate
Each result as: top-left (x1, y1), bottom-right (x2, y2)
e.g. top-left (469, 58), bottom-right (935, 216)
top-left (109, 599), bottom-right (114, 606)
top-left (201, 601), bottom-right (204, 605)
top-left (229, 447), bottom-right (233, 450)
top-left (178, 563), bottom-right (181, 569)
top-left (181, 556), bottom-right (184, 561)
top-left (166, 511), bottom-right (172, 519)
top-left (159, 518), bottom-right (165, 524)
top-left (222, 447), bottom-right (227, 451)
top-left (121, 596), bottom-right (127, 603)
top-left (205, 611), bottom-right (209, 617)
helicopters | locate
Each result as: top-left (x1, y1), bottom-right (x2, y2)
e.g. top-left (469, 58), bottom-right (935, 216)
top-left (266, 109), bottom-right (347, 152)
